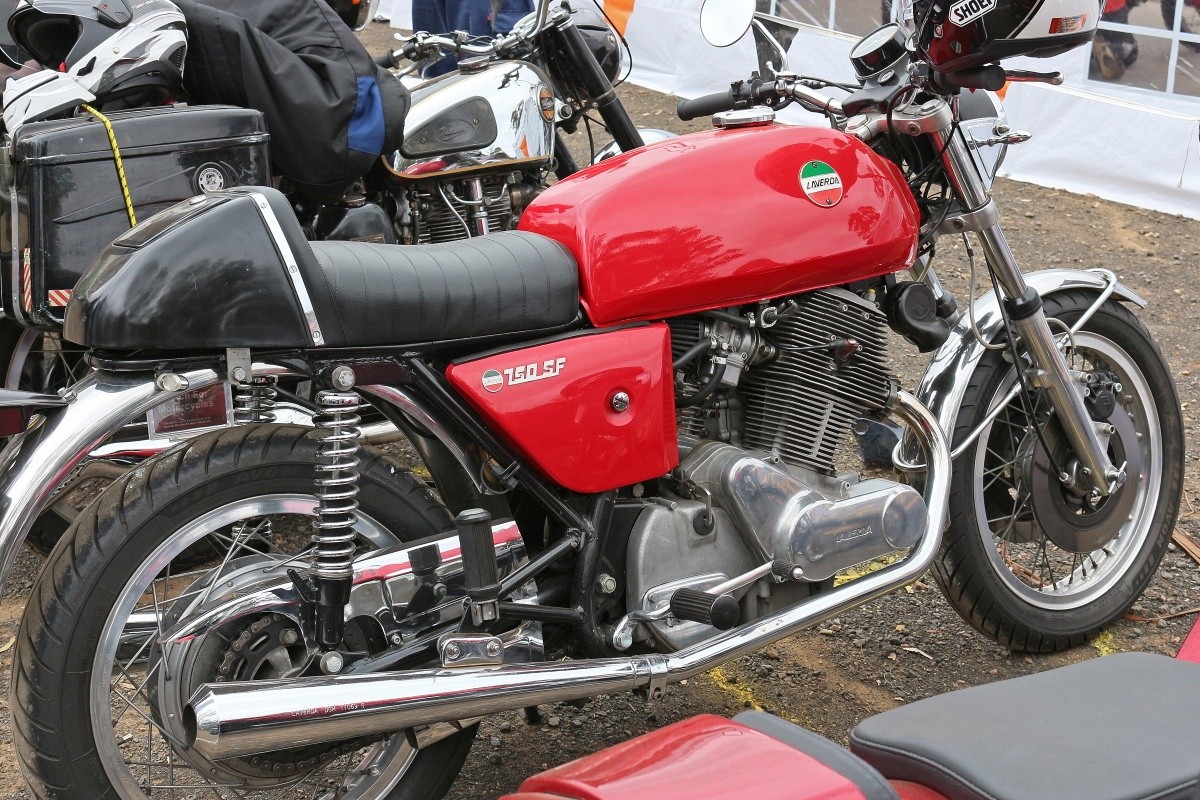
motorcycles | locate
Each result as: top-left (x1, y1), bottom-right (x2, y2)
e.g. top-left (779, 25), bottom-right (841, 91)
top-left (0, 0), bottom-right (1200, 800)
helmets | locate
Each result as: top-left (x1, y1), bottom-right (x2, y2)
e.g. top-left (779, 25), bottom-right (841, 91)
top-left (7, 0), bottom-right (189, 98)
